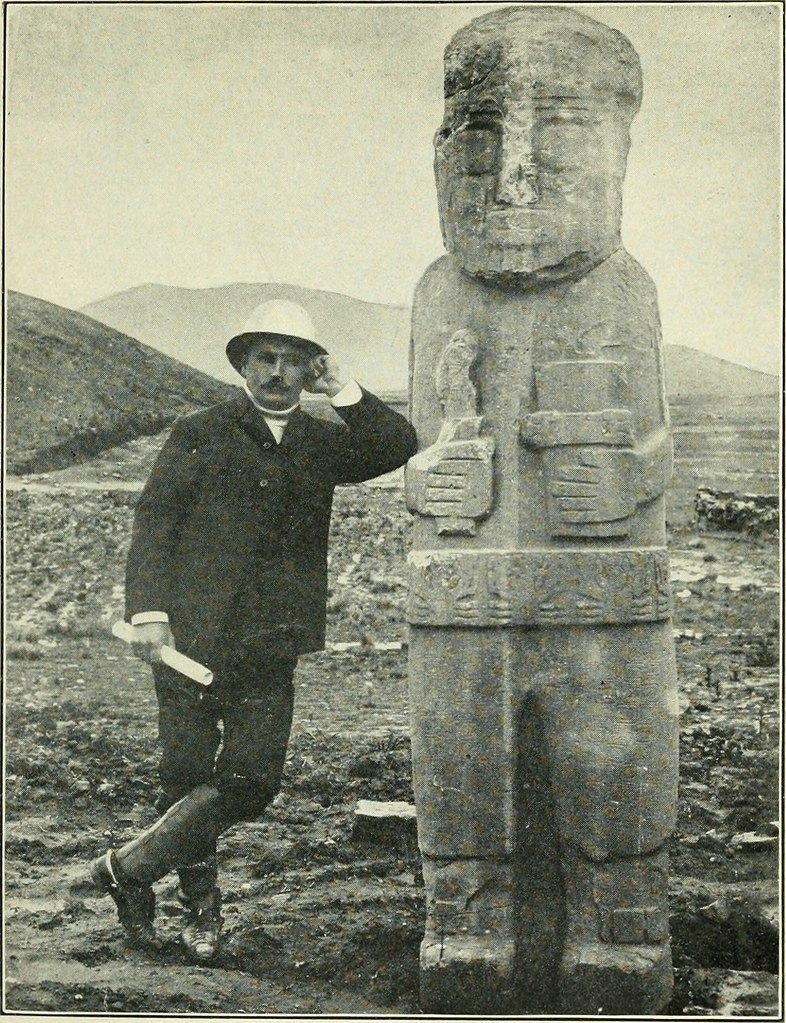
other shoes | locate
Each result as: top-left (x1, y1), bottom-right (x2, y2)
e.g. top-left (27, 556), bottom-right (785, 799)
top-left (91, 849), bottom-right (164, 952)
top-left (181, 906), bottom-right (223, 961)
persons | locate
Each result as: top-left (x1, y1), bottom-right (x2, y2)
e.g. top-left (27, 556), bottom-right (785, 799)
top-left (91, 297), bottom-right (417, 956)
top-left (407, 8), bottom-right (679, 1018)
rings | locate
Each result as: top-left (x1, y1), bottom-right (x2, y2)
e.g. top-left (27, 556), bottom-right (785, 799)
top-left (137, 651), bottom-right (143, 653)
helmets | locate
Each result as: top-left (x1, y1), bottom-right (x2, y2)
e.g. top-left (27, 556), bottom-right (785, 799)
top-left (226, 300), bottom-right (331, 388)
